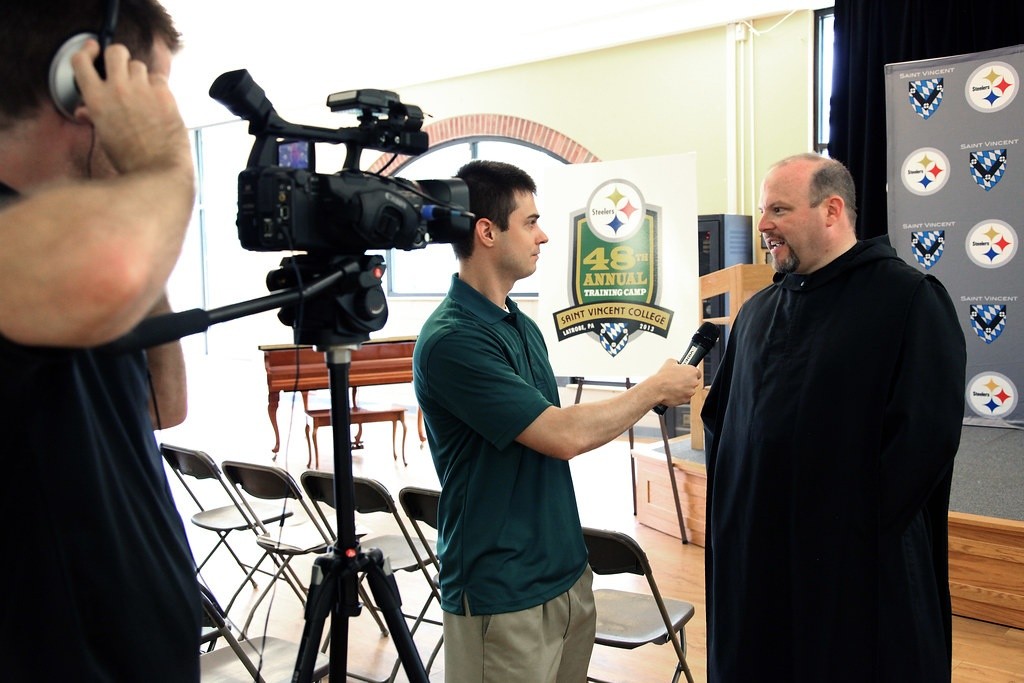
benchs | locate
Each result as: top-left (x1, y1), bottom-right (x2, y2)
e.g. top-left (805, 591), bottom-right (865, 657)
top-left (306, 404), bottom-right (408, 470)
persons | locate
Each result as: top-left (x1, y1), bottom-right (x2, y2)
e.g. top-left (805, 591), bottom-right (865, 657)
top-left (700, 154), bottom-right (966, 682)
top-left (0, 2), bottom-right (202, 683)
top-left (412, 161), bottom-right (702, 683)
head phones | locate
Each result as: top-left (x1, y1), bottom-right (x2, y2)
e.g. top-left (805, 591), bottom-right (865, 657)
top-left (46, 0), bottom-right (120, 123)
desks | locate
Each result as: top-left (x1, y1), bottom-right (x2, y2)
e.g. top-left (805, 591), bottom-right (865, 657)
top-left (256, 336), bottom-right (429, 455)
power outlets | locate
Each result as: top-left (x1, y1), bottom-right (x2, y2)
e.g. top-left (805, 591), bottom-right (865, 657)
top-left (735, 24), bottom-right (747, 41)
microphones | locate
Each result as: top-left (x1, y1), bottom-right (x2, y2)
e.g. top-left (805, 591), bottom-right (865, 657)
top-left (651, 321), bottom-right (721, 415)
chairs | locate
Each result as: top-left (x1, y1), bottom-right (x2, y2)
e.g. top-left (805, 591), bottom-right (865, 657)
top-left (301, 470), bottom-right (444, 683)
top-left (221, 461), bottom-right (389, 653)
top-left (398, 487), bottom-right (444, 676)
top-left (577, 526), bottom-right (695, 683)
top-left (197, 582), bottom-right (332, 683)
top-left (160, 443), bottom-right (310, 619)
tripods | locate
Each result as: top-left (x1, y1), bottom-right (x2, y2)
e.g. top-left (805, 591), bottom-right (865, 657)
top-left (116, 252), bottom-right (431, 683)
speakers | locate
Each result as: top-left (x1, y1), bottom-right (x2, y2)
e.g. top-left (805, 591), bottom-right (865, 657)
top-left (698, 214), bottom-right (752, 390)
